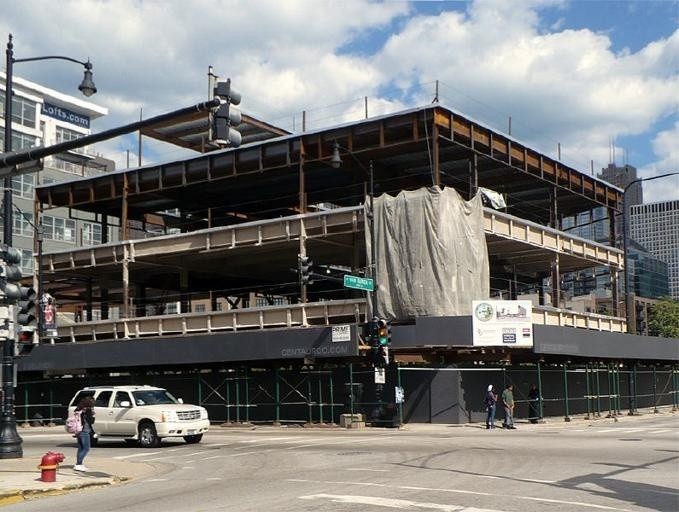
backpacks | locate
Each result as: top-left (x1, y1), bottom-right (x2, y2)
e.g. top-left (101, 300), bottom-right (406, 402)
top-left (66, 408), bottom-right (86, 434)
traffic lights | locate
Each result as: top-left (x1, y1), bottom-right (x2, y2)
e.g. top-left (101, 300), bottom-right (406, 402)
top-left (299, 255), bottom-right (315, 285)
top-left (1, 244), bottom-right (36, 356)
top-left (365, 319), bottom-right (392, 367)
top-left (213, 77), bottom-right (242, 147)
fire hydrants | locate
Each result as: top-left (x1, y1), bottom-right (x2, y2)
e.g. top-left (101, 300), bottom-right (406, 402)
top-left (38, 451), bottom-right (65, 482)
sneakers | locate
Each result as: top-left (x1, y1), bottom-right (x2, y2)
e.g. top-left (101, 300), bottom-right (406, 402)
top-left (73, 465), bottom-right (90, 472)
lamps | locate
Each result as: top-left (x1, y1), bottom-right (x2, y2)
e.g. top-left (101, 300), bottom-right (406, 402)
top-left (481, 347), bottom-right (506, 355)
top-left (587, 358), bottom-right (604, 365)
top-left (567, 357), bottom-right (572, 363)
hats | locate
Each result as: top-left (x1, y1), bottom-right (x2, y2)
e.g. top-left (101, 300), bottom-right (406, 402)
top-left (487, 384), bottom-right (493, 391)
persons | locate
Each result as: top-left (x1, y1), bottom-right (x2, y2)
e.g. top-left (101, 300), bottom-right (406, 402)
top-left (40, 288), bottom-right (59, 331)
top-left (72, 395), bottom-right (96, 472)
top-left (485, 384), bottom-right (498, 429)
top-left (501, 383), bottom-right (517, 429)
top-left (528, 383), bottom-right (540, 424)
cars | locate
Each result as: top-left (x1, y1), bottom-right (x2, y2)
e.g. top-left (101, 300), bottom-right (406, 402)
top-left (66, 384), bottom-right (210, 447)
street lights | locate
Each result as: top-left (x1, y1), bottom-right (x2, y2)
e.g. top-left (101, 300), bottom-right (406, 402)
top-left (330, 139), bottom-right (392, 427)
top-left (0, 33), bottom-right (97, 458)
